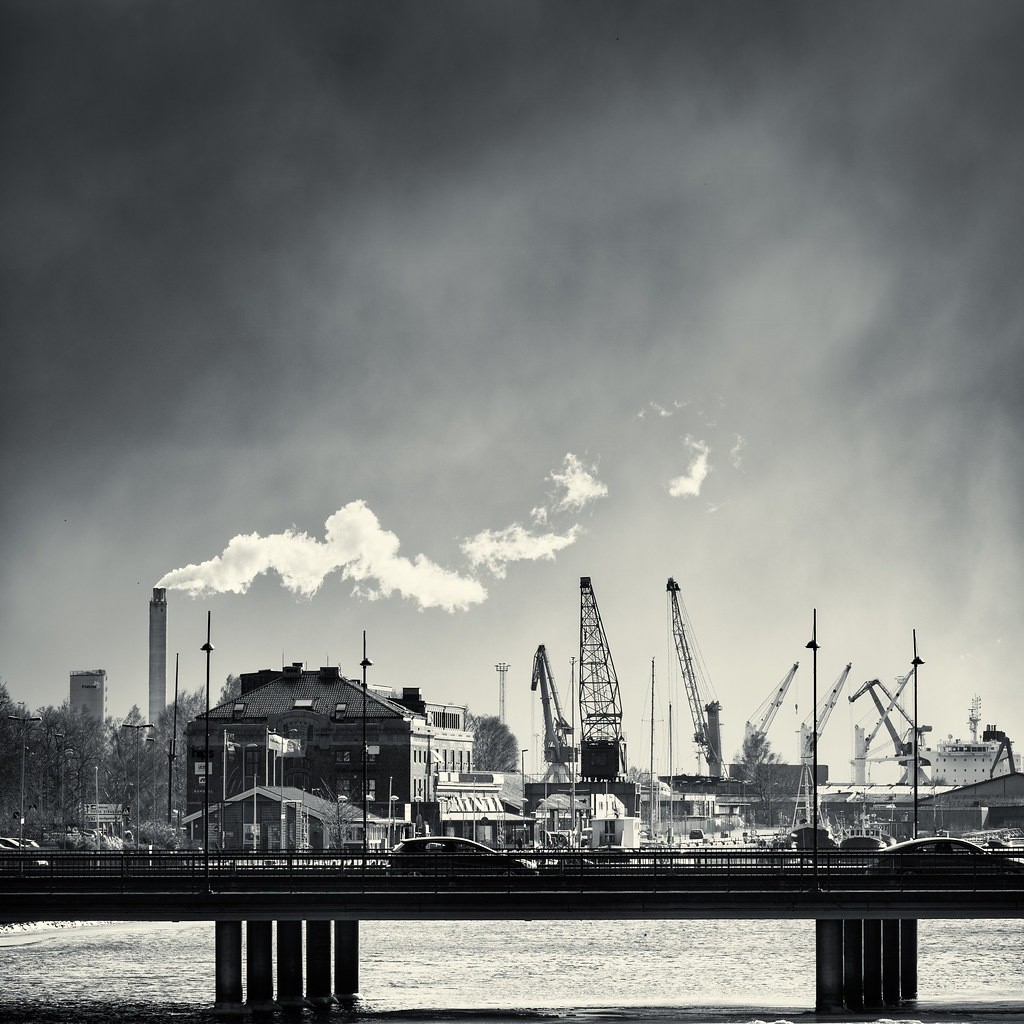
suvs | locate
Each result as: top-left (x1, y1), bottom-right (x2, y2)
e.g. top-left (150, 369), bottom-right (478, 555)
top-left (383, 835), bottom-right (538, 878)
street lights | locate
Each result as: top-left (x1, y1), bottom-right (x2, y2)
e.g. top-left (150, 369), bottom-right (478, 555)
top-left (200, 611), bottom-right (216, 888)
top-left (219, 727), bottom-right (589, 858)
top-left (358, 630), bottom-right (375, 874)
top-left (8, 713), bottom-right (158, 878)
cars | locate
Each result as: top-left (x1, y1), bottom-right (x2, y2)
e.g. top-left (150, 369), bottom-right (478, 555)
top-left (869, 837), bottom-right (1024, 878)
top-left (0, 834), bottom-right (40, 852)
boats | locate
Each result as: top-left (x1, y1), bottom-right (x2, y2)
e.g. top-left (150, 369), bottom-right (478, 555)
top-left (927, 695), bottom-right (1024, 787)
top-left (544, 825), bottom-right (1024, 858)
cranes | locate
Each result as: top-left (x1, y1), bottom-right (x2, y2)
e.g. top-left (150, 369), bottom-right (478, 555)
top-left (519, 573), bottom-right (936, 832)
